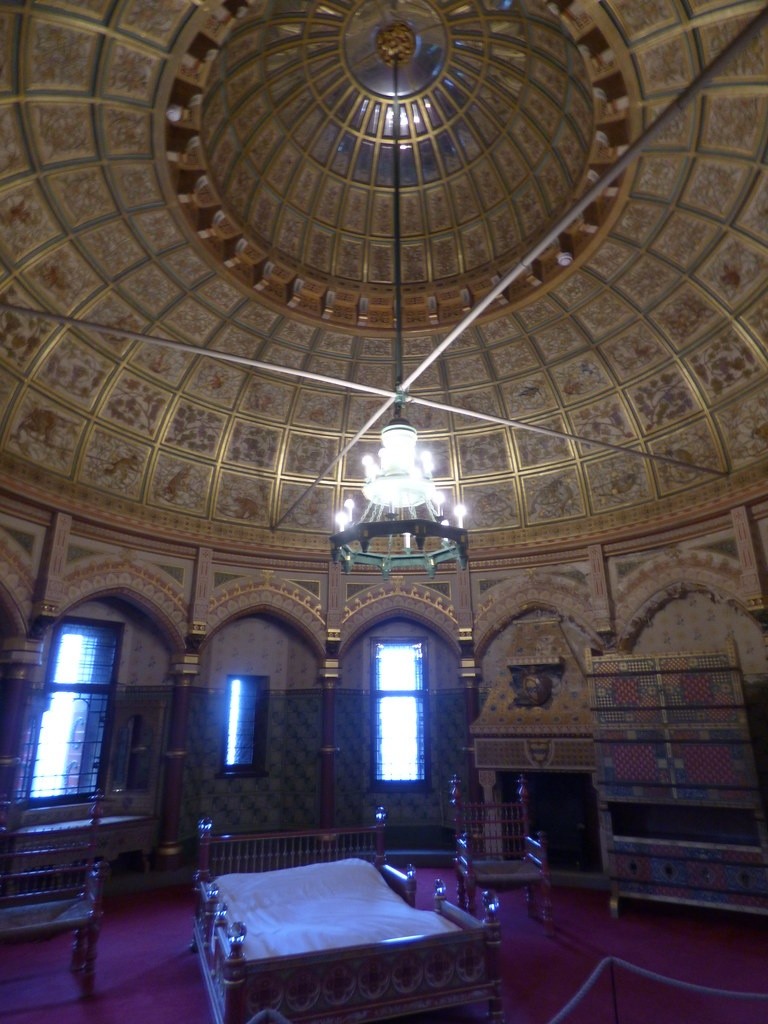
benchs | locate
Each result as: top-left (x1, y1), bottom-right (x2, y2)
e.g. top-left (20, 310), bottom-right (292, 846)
top-left (0, 787), bottom-right (111, 985)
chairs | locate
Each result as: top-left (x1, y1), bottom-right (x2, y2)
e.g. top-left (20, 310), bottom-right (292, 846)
top-left (448, 770), bottom-right (558, 941)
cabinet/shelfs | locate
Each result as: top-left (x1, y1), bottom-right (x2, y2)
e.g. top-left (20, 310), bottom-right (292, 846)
top-left (583, 634), bottom-right (768, 921)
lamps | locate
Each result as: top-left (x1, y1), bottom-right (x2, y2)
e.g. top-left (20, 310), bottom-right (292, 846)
top-left (327, 57), bottom-right (472, 582)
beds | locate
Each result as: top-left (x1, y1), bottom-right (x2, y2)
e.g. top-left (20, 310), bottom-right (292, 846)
top-left (190, 807), bottom-right (503, 1024)
